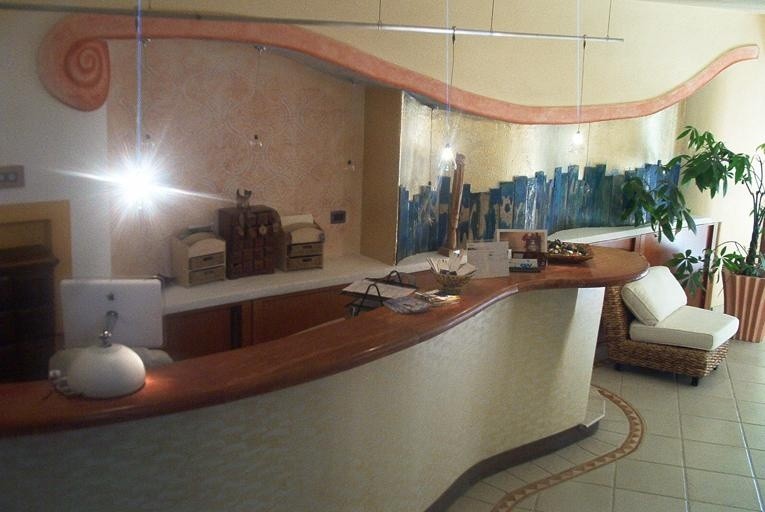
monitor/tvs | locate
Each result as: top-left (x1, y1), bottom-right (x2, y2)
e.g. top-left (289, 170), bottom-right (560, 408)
top-left (60, 278), bottom-right (164, 399)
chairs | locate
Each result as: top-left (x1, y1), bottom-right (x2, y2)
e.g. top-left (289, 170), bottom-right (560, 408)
top-left (602, 285), bottom-right (740, 386)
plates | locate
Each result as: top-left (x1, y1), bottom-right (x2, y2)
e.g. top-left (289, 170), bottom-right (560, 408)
top-left (547, 240), bottom-right (595, 263)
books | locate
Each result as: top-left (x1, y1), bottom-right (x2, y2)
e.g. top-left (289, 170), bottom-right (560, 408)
top-left (380, 288), bottom-right (461, 315)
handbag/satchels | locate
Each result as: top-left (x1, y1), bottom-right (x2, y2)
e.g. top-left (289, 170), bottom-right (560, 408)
top-left (344, 269), bottom-right (420, 316)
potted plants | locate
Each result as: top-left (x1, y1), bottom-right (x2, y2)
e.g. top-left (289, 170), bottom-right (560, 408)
top-left (619, 121), bottom-right (765, 343)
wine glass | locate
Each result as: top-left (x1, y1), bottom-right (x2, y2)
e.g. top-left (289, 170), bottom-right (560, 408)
top-left (430, 266), bottom-right (478, 295)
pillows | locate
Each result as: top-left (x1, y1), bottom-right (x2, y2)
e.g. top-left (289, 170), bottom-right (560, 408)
top-left (621, 265), bottom-right (689, 324)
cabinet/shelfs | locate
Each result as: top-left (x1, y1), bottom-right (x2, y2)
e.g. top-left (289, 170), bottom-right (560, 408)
top-left (159, 269), bottom-right (439, 362)
